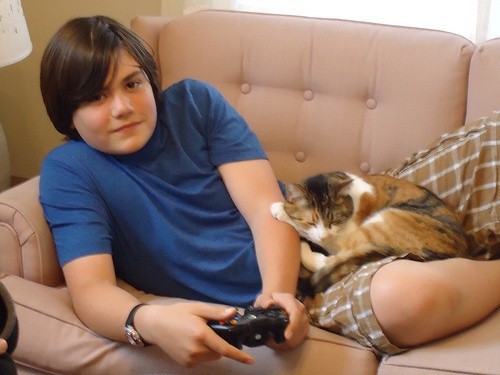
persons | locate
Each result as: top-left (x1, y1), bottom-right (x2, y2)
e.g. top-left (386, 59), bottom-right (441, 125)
top-left (39, 16), bottom-right (500, 369)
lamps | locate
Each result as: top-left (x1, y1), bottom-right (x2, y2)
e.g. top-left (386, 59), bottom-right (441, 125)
top-left (0, 0), bottom-right (34, 194)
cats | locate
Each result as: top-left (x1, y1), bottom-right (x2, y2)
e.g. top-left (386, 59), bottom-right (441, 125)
top-left (271, 171), bottom-right (490, 298)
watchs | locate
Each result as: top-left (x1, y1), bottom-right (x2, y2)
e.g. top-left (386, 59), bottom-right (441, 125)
top-left (124, 303), bottom-right (153, 347)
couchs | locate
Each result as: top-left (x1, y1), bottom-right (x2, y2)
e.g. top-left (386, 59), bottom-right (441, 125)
top-left (0, 8), bottom-right (500, 375)
